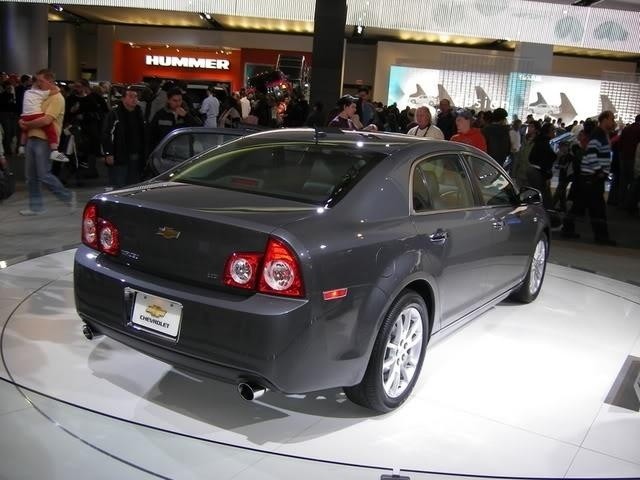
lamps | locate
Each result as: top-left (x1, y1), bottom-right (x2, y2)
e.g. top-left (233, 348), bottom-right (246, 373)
top-left (352, 25), bottom-right (365, 37)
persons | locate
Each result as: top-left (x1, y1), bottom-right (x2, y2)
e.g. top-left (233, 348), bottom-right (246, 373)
top-left (99, 83), bottom-right (312, 188)
top-left (327, 87), bottom-right (640, 245)
top-left (0, 70), bottom-right (112, 215)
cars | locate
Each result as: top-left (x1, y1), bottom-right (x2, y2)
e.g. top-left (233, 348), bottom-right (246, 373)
top-left (502, 132), bottom-right (613, 193)
top-left (247, 70), bottom-right (292, 100)
top-left (408, 95), bottom-right (562, 117)
top-left (71, 124), bottom-right (551, 415)
top-left (139, 126), bottom-right (282, 184)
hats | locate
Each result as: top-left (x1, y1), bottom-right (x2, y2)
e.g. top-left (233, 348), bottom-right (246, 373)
top-left (337, 93), bottom-right (359, 107)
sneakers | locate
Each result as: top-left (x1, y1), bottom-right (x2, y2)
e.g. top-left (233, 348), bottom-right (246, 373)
top-left (19, 209), bottom-right (45, 215)
top-left (48, 151), bottom-right (70, 163)
top-left (17, 146), bottom-right (27, 159)
top-left (67, 191), bottom-right (77, 216)
